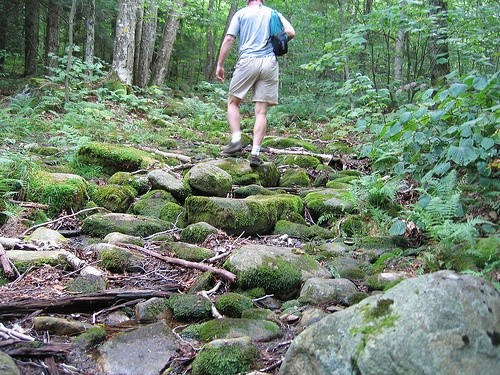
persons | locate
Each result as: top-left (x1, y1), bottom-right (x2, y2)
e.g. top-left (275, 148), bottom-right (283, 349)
top-left (215, 0), bottom-right (296, 166)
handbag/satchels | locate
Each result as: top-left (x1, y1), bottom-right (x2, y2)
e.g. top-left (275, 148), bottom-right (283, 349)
top-left (270, 9), bottom-right (288, 55)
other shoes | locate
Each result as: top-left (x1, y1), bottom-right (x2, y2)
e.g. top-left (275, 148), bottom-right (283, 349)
top-left (221, 141), bottom-right (242, 156)
top-left (249, 154), bottom-right (264, 167)
